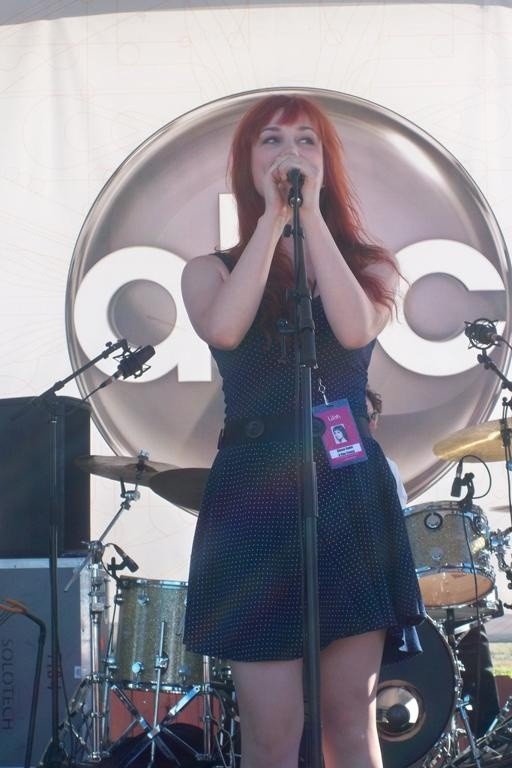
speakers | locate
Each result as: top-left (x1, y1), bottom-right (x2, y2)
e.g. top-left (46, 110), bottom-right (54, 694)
top-left (0, 395), bottom-right (92, 558)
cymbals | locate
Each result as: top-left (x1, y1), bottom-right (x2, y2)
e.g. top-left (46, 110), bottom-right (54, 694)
top-left (73, 454), bottom-right (180, 487)
top-left (433, 419), bottom-right (512, 463)
top-left (148, 468), bottom-right (211, 510)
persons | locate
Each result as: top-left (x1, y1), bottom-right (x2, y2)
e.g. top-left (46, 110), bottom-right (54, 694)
top-left (180, 92), bottom-right (426, 767)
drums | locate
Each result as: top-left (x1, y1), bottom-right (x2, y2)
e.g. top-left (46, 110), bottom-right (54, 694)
top-left (401, 499), bottom-right (494, 607)
top-left (421, 587), bottom-right (501, 622)
top-left (111, 574), bottom-right (205, 695)
top-left (374, 614), bottom-right (464, 768)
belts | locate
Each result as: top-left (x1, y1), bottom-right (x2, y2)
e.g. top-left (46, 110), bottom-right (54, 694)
top-left (217, 417), bottom-right (371, 450)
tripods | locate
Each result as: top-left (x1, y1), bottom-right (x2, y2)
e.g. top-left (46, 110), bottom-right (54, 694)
top-left (442, 609), bottom-right (482, 768)
top-left (37, 500), bottom-right (180, 768)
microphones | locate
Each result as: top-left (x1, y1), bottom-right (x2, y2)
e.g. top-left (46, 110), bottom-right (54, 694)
top-left (451, 458), bottom-right (463, 497)
top-left (100, 344), bottom-right (156, 389)
top-left (287, 171), bottom-right (304, 208)
top-left (464, 324), bottom-right (502, 344)
